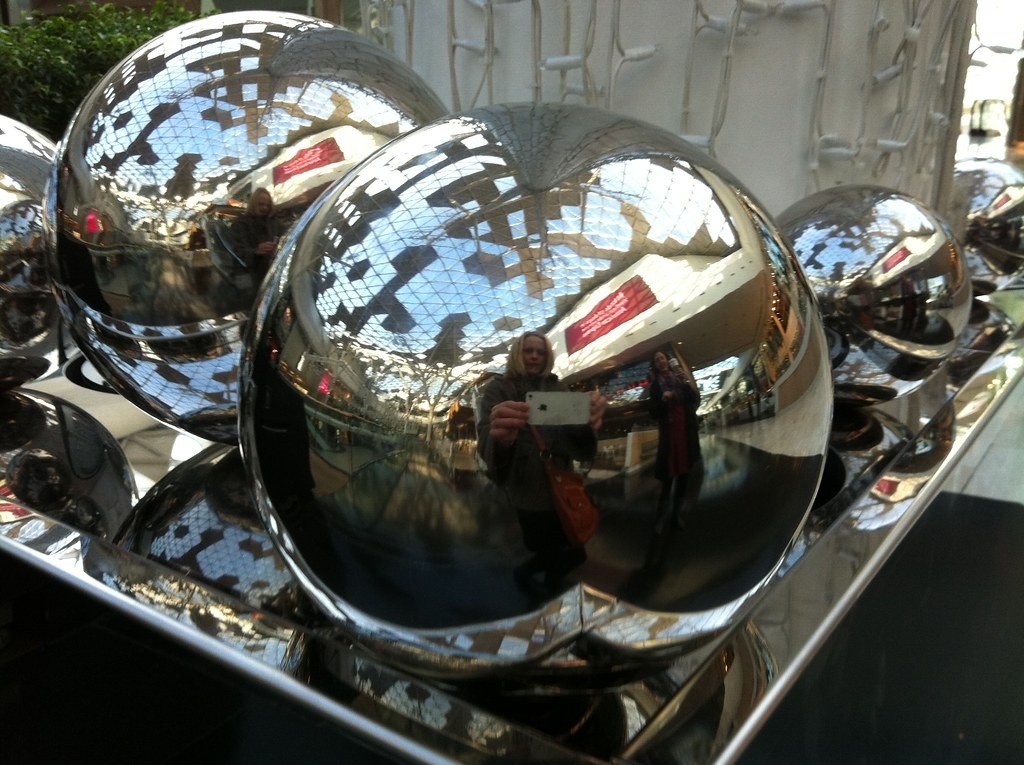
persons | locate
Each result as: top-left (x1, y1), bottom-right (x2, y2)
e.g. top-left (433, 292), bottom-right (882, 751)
top-left (184, 224), bottom-right (206, 250)
top-left (476, 331), bottom-right (605, 597)
top-left (230, 188), bottom-right (280, 289)
top-left (648, 349), bottom-right (704, 520)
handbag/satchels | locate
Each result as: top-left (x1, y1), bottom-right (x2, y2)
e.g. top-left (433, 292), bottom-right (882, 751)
top-left (548, 468), bottom-right (599, 544)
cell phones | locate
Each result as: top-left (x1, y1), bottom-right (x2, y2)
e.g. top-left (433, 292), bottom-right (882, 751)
top-left (526, 391), bottom-right (591, 426)
